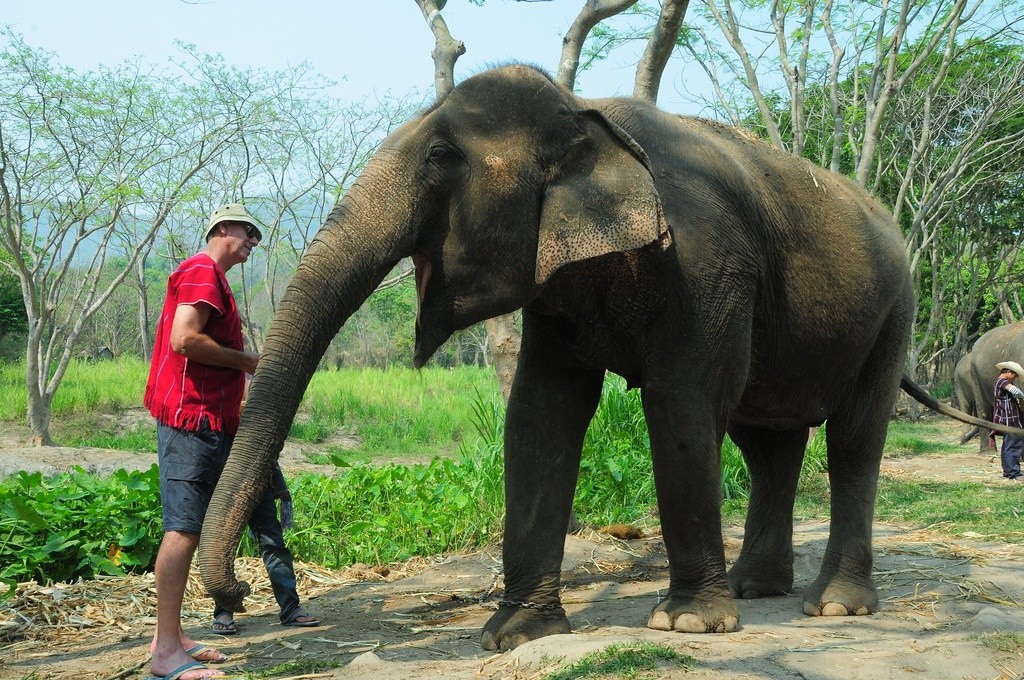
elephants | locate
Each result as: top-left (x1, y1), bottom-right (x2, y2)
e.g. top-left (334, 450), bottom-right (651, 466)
top-left (953, 319), bottom-right (1024, 457)
top-left (196, 60), bottom-right (921, 655)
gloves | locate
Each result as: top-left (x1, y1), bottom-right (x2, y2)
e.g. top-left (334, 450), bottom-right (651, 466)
top-left (281, 499), bottom-right (292, 529)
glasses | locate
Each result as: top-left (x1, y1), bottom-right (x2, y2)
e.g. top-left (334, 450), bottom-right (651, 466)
top-left (234, 221), bottom-right (256, 238)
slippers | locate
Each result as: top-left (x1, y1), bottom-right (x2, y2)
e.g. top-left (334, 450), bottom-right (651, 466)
top-left (185, 644), bottom-right (227, 663)
top-left (213, 612), bottom-right (236, 633)
top-left (282, 612), bottom-right (320, 626)
top-left (147, 661), bottom-right (225, 680)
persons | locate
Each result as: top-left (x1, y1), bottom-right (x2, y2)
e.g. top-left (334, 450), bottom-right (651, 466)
top-left (143, 204), bottom-right (261, 680)
top-left (211, 377), bottom-right (320, 634)
top-left (989, 361), bottom-right (1024, 479)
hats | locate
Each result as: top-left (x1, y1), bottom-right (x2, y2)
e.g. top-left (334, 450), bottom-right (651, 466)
top-left (996, 361), bottom-right (1024, 382)
top-left (204, 203), bottom-right (263, 244)
